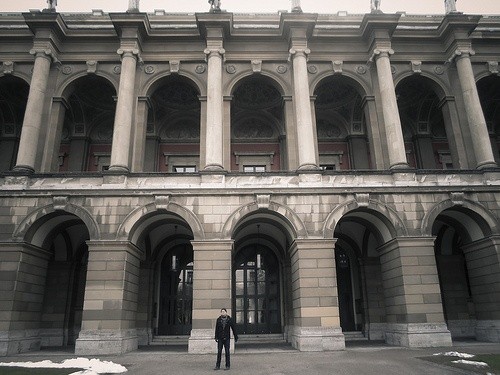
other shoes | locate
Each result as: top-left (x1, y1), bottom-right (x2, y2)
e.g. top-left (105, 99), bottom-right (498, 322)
top-left (213, 367), bottom-right (220, 370)
top-left (223, 367), bottom-right (229, 370)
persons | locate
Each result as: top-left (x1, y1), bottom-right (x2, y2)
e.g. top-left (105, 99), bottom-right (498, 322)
top-left (213, 307), bottom-right (238, 370)
